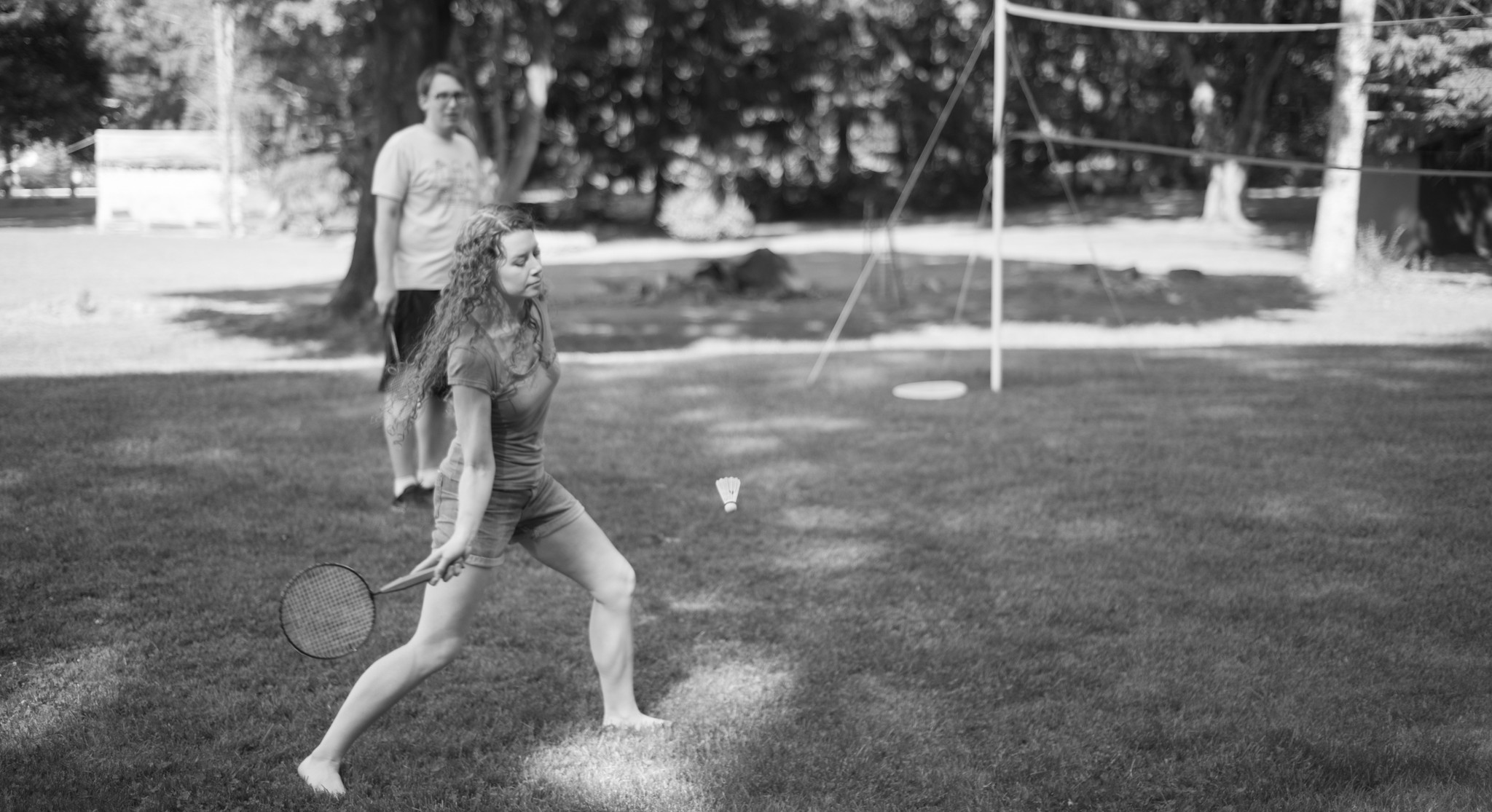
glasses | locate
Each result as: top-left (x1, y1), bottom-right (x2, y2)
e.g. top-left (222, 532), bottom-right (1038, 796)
top-left (428, 92), bottom-right (470, 105)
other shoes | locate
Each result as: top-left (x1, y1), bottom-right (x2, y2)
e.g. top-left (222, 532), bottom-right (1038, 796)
top-left (391, 482), bottom-right (435, 513)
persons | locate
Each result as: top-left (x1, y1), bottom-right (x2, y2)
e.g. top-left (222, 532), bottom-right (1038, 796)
top-left (297, 208), bottom-right (681, 800)
top-left (372, 63), bottom-right (492, 509)
